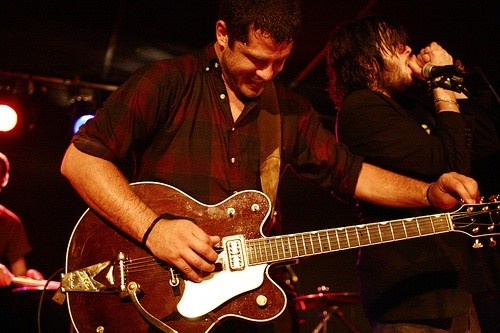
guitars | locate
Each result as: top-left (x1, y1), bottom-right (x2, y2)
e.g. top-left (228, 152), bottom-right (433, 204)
top-left (52, 181), bottom-right (500, 333)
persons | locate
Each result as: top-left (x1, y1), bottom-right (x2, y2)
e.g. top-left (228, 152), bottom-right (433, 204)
top-left (326, 15), bottom-right (481, 333)
top-left (0, 151), bottom-right (44, 286)
top-left (59, 0), bottom-right (480, 332)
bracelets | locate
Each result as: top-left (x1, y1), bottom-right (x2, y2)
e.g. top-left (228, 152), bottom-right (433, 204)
top-left (431, 97), bottom-right (460, 108)
top-left (142, 215), bottom-right (164, 243)
top-left (425, 183), bottom-right (433, 207)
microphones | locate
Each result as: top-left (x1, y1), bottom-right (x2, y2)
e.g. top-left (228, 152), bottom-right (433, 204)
top-left (421, 62), bottom-right (467, 81)
